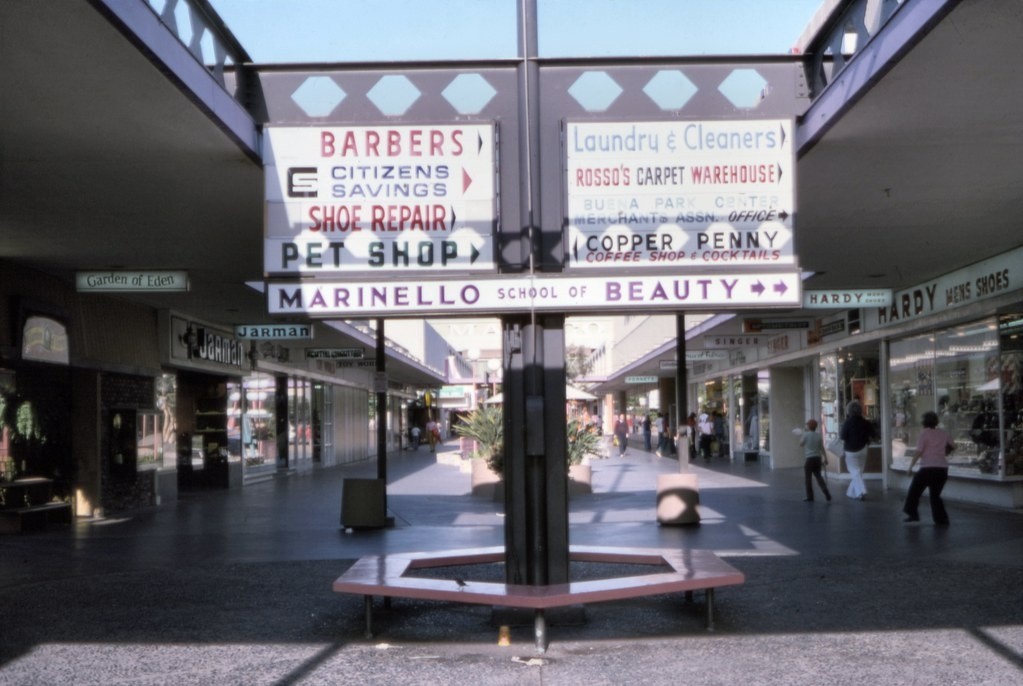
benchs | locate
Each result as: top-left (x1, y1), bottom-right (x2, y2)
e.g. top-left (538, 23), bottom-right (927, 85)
top-left (733, 451), bottom-right (758, 464)
top-left (333, 545), bottom-right (749, 653)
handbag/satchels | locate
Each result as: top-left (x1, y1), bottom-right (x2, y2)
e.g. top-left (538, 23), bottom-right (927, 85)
top-left (826, 437), bottom-right (845, 458)
top-left (711, 435), bottom-right (716, 442)
top-left (613, 438), bottom-right (619, 447)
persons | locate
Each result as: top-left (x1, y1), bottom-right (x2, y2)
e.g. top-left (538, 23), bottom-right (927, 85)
top-left (632, 414), bottom-right (640, 433)
top-left (655, 412), bottom-right (675, 458)
top-left (410, 422), bottom-right (422, 450)
top-left (426, 417), bottom-right (442, 452)
top-left (901, 411), bottom-right (959, 529)
top-left (686, 411), bottom-right (699, 458)
top-left (840, 399), bottom-right (875, 500)
top-left (643, 415), bottom-right (653, 451)
top-left (615, 412), bottom-right (629, 458)
top-left (697, 404), bottom-right (728, 460)
top-left (596, 415), bottom-right (603, 436)
top-left (797, 418), bottom-right (834, 502)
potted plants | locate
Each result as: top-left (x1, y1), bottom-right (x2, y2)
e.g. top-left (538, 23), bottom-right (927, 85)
top-left (453, 401), bottom-right (611, 504)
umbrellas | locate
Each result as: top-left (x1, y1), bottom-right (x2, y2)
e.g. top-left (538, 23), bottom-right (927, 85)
top-left (481, 379), bottom-right (599, 404)
top-left (227, 407), bottom-right (273, 424)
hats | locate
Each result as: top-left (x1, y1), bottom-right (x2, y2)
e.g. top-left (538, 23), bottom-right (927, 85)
top-left (805, 419), bottom-right (817, 428)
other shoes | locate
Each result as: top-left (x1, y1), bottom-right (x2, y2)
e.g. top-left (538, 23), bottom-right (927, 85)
top-left (803, 498), bottom-right (814, 502)
top-left (933, 521), bottom-right (950, 529)
top-left (859, 494), bottom-right (865, 501)
top-left (903, 516), bottom-right (920, 522)
top-left (826, 496), bottom-right (831, 501)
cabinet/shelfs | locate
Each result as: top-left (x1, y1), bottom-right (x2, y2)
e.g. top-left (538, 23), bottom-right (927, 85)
top-left (951, 409), bottom-right (1009, 432)
top-left (194, 413), bottom-right (227, 432)
